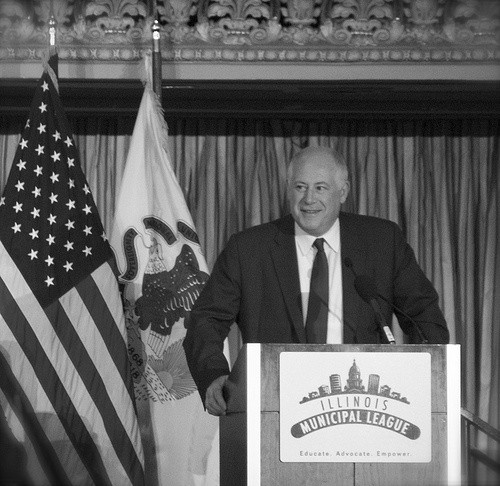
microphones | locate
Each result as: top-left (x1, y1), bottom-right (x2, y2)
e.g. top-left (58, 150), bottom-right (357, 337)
top-left (344, 257), bottom-right (428, 344)
top-left (353, 275), bottom-right (395, 344)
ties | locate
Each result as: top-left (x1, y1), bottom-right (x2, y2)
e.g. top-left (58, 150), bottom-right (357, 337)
top-left (305, 238), bottom-right (329, 344)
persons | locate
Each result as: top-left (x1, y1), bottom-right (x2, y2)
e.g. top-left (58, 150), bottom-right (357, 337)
top-left (183, 146), bottom-right (449, 417)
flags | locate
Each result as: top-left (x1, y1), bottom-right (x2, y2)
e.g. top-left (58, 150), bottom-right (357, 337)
top-left (0, 46), bottom-right (146, 486)
top-left (108, 88), bottom-right (231, 486)
